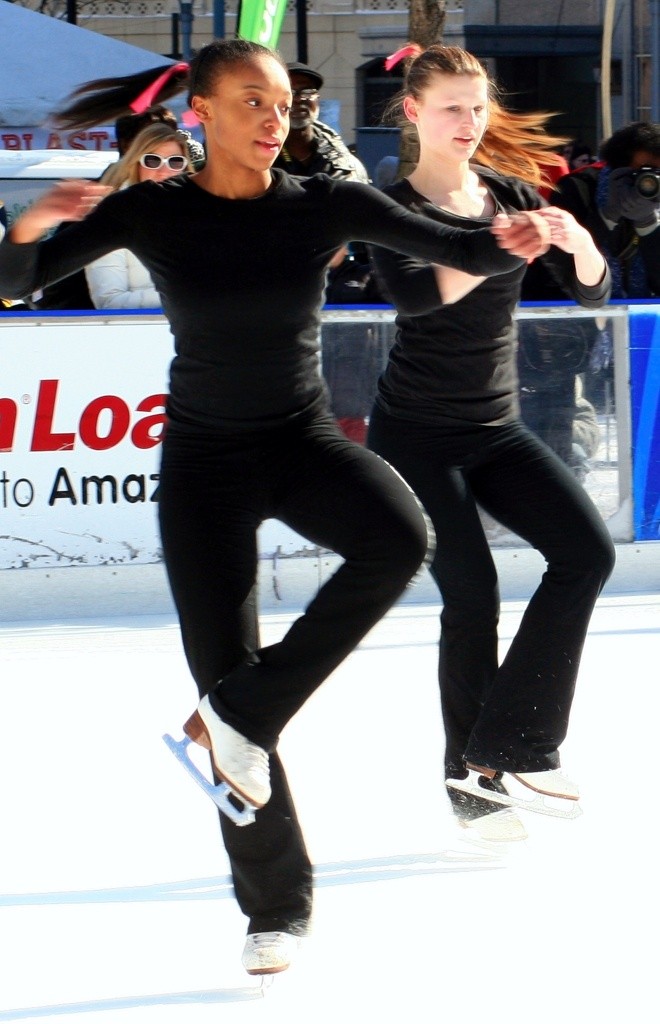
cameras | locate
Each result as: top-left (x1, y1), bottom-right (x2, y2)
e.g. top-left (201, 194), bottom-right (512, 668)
top-left (622, 166), bottom-right (660, 206)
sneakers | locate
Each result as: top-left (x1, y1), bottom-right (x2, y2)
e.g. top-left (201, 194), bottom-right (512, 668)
top-left (162, 694), bottom-right (274, 829)
top-left (453, 811), bottom-right (530, 857)
top-left (240, 929), bottom-right (311, 1001)
top-left (445, 758), bottom-right (584, 819)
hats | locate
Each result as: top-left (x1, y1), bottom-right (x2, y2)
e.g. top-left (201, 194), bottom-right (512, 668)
top-left (284, 62), bottom-right (324, 91)
top-left (570, 143), bottom-right (591, 161)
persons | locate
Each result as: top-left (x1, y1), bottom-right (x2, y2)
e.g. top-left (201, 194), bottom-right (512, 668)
top-left (350, 121), bottom-right (660, 302)
top-left (365, 44), bottom-right (616, 842)
top-left (232, 62), bottom-right (370, 308)
top-left (72, 123), bottom-right (195, 312)
top-left (0, 41), bottom-right (547, 977)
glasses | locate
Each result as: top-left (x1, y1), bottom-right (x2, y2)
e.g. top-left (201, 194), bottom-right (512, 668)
top-left (134, 152), bottom-right (189, 172)
top-left (288, 86), bottom-right (320, 101)
top-left (577, 159), bottom-right (591, 163)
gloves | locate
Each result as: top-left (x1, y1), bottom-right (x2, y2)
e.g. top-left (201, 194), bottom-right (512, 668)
top-left (619, 192), bottom-right (659, 225)
top-left (603, 166), bottom-right (633, 222)
